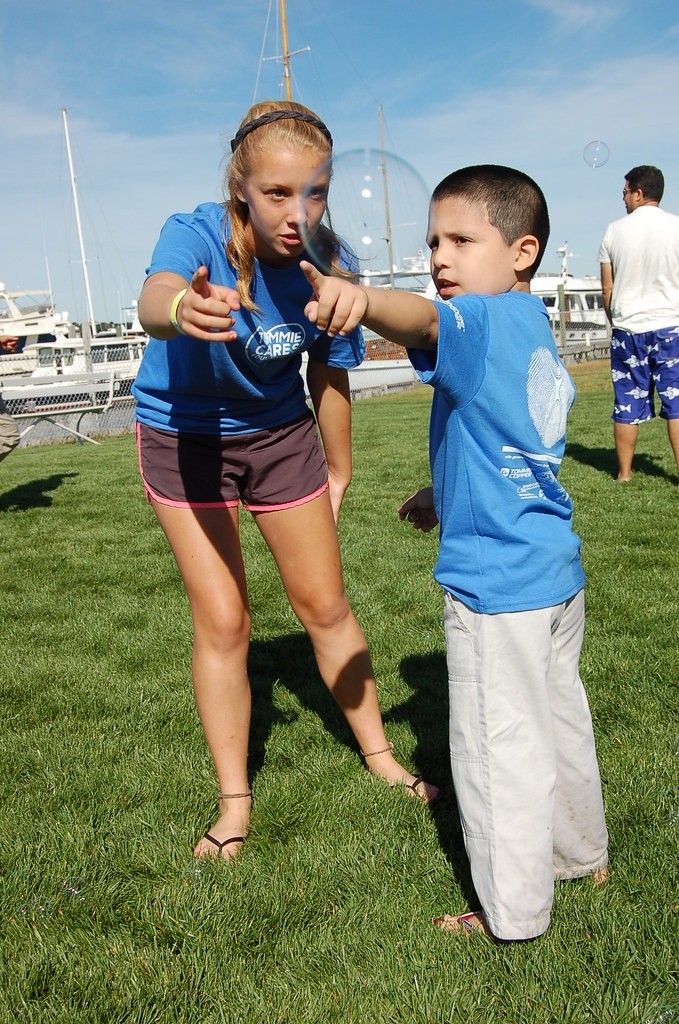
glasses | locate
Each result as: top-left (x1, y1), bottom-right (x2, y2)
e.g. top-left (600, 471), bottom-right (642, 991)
top-left (623, 190), bottom-right (632, 194)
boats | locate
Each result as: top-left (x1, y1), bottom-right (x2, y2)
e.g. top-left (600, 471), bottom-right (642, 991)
top-left (0, 0), bottom-right (611, 418)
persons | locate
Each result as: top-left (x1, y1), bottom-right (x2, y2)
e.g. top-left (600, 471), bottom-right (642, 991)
top-left (300, 164), bottom-right (610, 942)
top-left (597, 166), bottom-right (679, 482)
top-left (0, 336), bottom-right (21, 461)
top-left (132, 101), bottom-right (439, 862)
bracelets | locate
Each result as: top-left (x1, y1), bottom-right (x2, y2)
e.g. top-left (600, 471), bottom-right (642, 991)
top-left (171, 289), bottom-right (186, 335)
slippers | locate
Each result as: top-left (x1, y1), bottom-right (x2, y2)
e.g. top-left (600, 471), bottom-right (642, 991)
top-left (193, 790), bottom-right (251, 865)
top-left (360, 744), bottom-right (438, 806)
top-left (581, 864), bottom-right (611, 895)
top-left (436, 909), bottom-right (543, 940)
